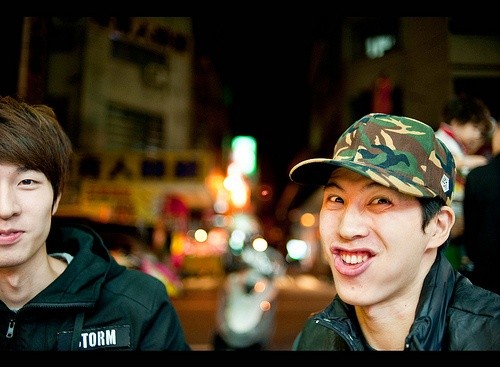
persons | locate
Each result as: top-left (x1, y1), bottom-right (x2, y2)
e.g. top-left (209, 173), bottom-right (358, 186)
top-left (434, 100), bottom-right (500, 296)
top-left (288, 113), bottom-right (500, 351)
top-left (0, 96), bottom-right (193, 352)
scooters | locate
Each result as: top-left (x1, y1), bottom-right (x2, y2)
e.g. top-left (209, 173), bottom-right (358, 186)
top-left (210, 235), bottom-right (292, 350)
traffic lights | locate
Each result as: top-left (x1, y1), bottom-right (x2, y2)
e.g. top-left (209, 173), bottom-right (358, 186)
top-left (224, 135), bottom-right (259, 179)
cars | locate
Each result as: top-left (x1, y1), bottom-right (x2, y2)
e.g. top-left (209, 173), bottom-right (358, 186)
top-left (99, 230), bottom-right (186, 301)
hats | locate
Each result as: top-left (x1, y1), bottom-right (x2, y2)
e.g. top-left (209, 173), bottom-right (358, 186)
top-left (288, 112), bottom-right (458, 206)
